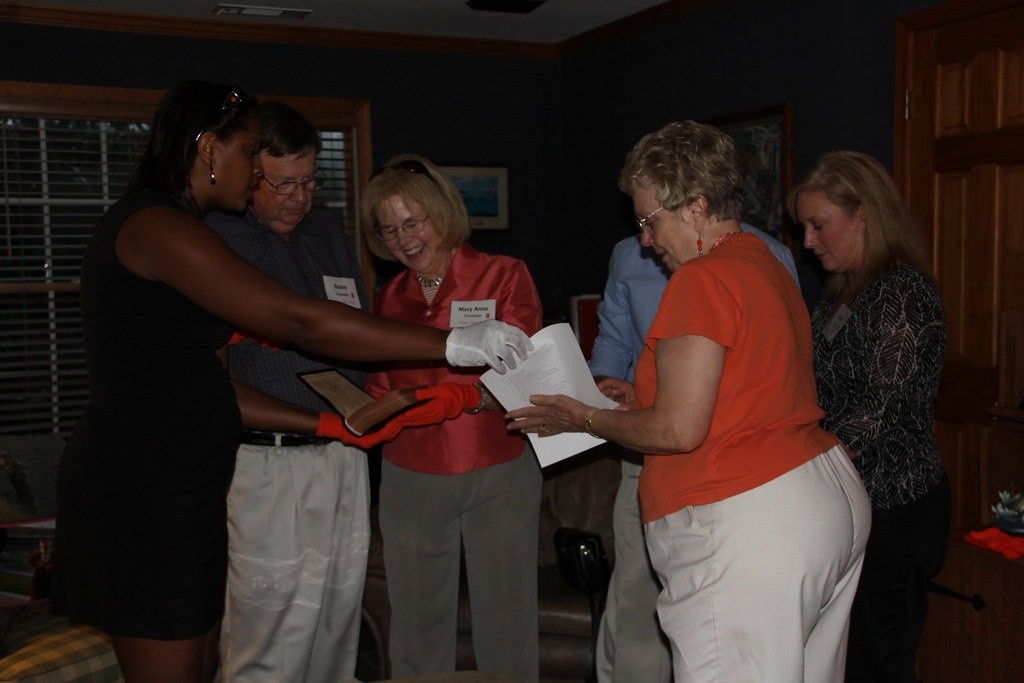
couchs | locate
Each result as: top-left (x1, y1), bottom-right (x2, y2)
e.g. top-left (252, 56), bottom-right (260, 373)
top-left (359, 454), bottom-right (621, 683)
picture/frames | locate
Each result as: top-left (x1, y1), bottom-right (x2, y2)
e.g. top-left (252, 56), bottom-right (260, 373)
top-left (694, 102), bottom-right (794, 261)
top-left (433, 161), bottom-right (515, 235)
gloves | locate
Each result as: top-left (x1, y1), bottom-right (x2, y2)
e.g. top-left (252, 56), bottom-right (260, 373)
top-left (401, 382), bottom-right (478, 428)
top-left (315, 408), bottom-right (403, 447)
top-left (447, 319), bottom-right (535, 374)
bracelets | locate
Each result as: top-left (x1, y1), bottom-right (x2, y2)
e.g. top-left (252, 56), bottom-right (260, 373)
top-left (584, 408), bottom-right (601, 438)
top-left (465, 383), bottom-right (487, 415)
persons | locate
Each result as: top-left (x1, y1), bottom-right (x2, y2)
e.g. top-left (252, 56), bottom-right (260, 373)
top-left (358, 152), bottom-right (544, 683)
top-left (502, 117), bottom-right (876, 683)
top-left (586, 135), bottom-right (798, 683)
top-left (785, 149), bottom-right (956, 683)
top-left (37, 76), bottom-right (534, 683)
top-left (195, 104), bottom-right (373, 682)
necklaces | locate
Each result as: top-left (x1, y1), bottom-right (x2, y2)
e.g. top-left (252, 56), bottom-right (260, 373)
top-left (415, 271), bottom-right (445, 287)
top-left (707, 231), bottom-right (735, 252)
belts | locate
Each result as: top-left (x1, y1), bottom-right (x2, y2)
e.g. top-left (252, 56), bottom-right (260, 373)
top-left (238, 429), bottom-right (335, 446)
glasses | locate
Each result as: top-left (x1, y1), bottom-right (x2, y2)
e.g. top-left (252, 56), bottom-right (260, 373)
top-left (377, 212), bottom-right (431, 241)
top-left (367, 160), bottom-right (440, 185)
top-left (262, 162), bottom-right (329, 195)
top-left (633, 203), bottom-right (672, 230)
top-left (194, 84), bottom-right (245, 142)
